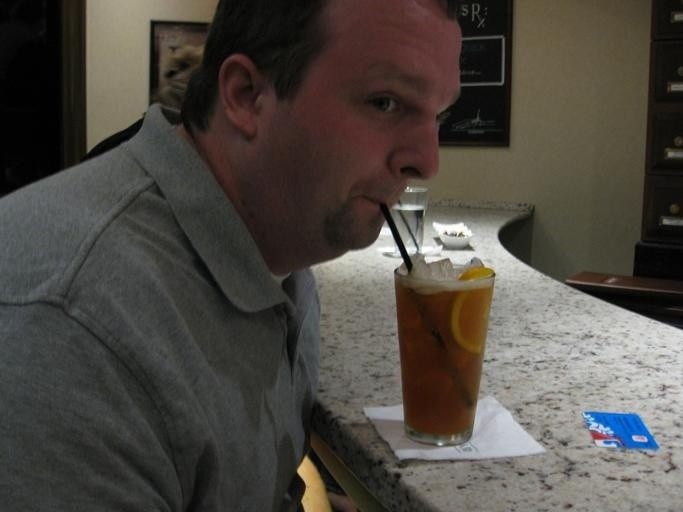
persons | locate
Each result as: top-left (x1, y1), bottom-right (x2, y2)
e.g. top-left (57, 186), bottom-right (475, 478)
top-left (0, 0), bottom-right (461, 512)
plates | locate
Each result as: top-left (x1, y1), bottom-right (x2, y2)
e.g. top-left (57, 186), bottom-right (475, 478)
top-left (434, 223), bottom-right (475, 249)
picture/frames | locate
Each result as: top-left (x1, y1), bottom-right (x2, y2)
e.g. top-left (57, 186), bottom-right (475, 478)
top-left (148, 20), bottom-right (210, 103)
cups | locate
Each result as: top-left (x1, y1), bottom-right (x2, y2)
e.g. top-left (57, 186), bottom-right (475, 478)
top-left (394, 263), bottom-right (496, 448)
top-left (392, 186), bottom-right (429, 257)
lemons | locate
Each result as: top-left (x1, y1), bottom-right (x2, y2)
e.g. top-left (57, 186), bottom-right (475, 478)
top-left (450, 267), bottom-right (496, 354)
